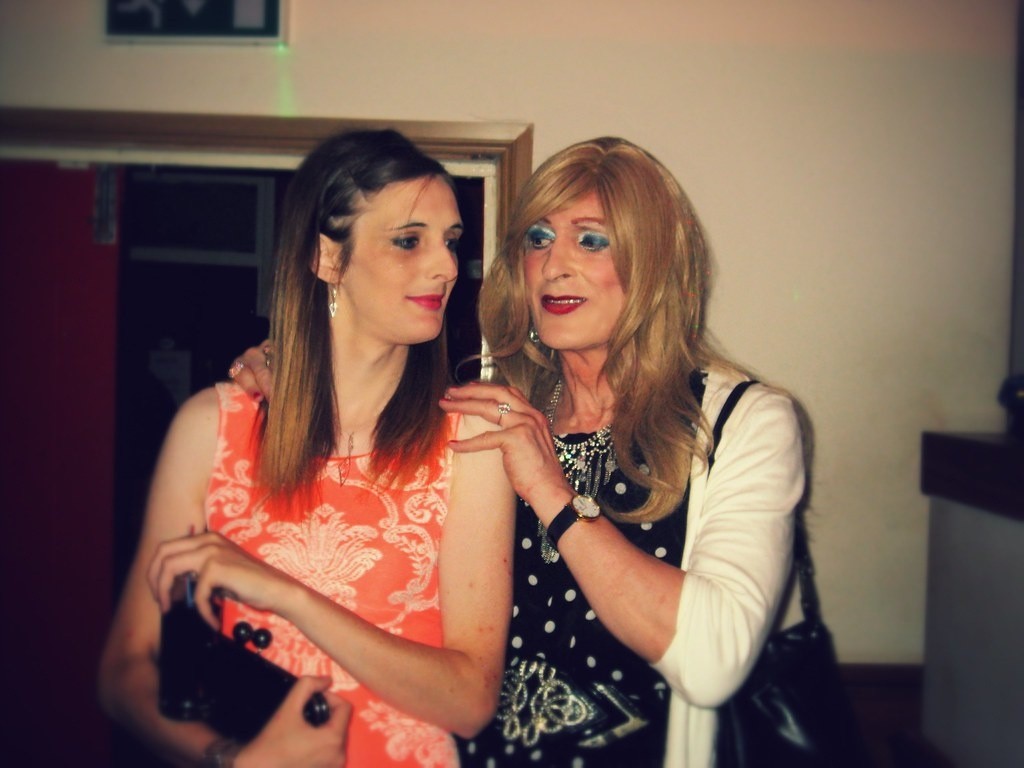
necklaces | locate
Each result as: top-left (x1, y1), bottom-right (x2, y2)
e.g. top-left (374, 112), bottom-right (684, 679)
top-left (337, 430), bottom-right (356, 487)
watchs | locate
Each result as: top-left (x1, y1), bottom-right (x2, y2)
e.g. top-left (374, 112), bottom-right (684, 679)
top-left (545, 493), bottom-right (601, 552)
top-left (200, 735), bottom-right (236, 768)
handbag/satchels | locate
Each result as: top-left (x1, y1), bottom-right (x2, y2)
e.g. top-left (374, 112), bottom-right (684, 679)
top-left (707, 380), bottom-right (874, 768)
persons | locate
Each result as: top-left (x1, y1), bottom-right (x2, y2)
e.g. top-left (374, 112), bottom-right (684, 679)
top-left (93, 128), bottom-right (518, 768)
top-left (229, 137), bottom-right (807, 768)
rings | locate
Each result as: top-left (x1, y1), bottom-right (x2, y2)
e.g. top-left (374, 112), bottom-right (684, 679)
top-left (263, 344), bottom-right (273, 367)
top-left (228, 360), bottom-right (245, 379)
top-left (497, 403), bottom-right (511, 426)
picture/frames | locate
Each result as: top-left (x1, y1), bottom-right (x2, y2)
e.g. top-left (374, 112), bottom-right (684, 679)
top-left (103, 0), bottom-right (289, 47)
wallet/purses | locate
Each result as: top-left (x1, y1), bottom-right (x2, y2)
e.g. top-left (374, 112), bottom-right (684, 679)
top-left (109, 601), bottom-right (330, 768)
